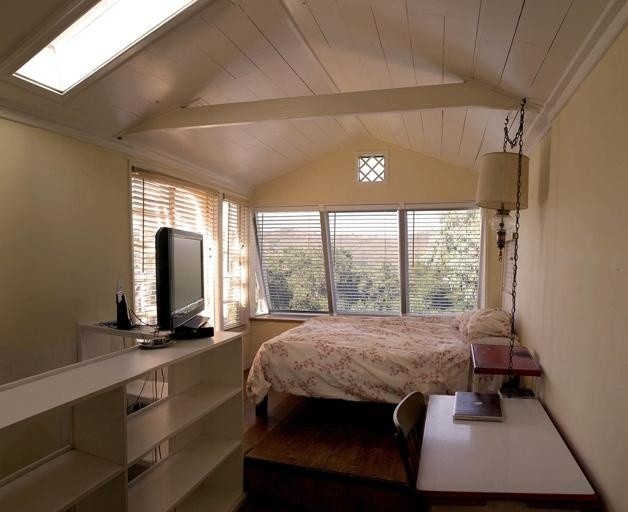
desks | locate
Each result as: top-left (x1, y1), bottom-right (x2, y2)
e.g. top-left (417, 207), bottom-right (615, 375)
top-left (417, 395), bottom-right (600, 507)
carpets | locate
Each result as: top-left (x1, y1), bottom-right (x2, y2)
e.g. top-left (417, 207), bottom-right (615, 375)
top-left (240, 456), bottom-right (430, 512)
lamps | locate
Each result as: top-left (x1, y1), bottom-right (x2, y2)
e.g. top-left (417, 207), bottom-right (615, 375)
top-left (475, 151), bottom-right (529, 261)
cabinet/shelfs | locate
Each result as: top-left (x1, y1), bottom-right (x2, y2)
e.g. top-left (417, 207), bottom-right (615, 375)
top-left (0, 318), bottom-right (247, 512)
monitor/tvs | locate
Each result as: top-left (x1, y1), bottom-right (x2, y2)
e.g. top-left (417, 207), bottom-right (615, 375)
top-left (155, 226), bottom-right (210, 331)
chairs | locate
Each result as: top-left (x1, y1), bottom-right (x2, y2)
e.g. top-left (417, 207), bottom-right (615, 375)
top-left (393, 391), bottom-right (426, 489)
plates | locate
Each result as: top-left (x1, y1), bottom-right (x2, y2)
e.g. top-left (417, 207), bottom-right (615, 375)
top-left (138, 340), bottom-right (177, 349)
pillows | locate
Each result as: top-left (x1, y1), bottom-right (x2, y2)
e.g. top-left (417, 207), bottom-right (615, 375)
top-left (452, 308), bottom-right (513, 339)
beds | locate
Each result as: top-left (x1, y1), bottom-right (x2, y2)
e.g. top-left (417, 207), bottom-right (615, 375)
top-left (247, 307), bottom-right (522, 418)
